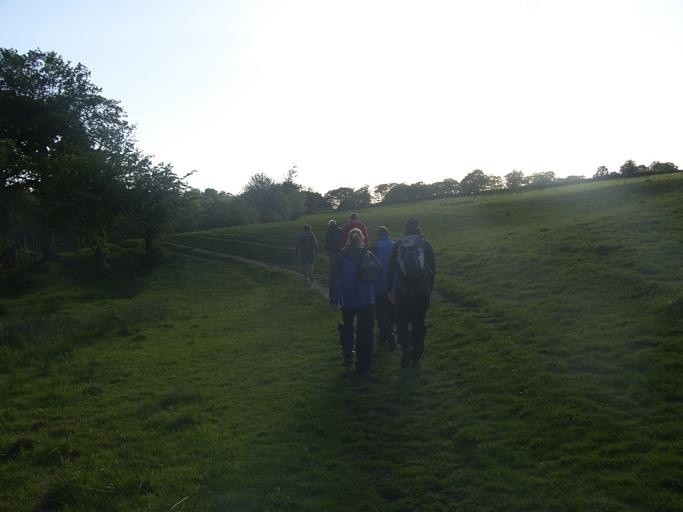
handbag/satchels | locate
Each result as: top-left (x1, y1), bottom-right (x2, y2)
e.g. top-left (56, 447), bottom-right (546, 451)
top-left (403, 271), bottom-right (433, 300)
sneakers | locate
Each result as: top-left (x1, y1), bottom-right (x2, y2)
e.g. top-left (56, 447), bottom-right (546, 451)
top-left (412, 359), bottom-right (420, 369)
top-left (355, 361), bottom-right (370, 375)
top-left (341, 357), bottom-right (354, 368)
top-left (400, 349), bottom-right (413, 368)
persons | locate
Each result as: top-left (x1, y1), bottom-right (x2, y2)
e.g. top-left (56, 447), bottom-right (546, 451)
top-left (292, 212), bottom-right (438, 376)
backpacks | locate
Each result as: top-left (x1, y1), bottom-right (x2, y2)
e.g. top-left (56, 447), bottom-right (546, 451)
top-left (356, 249), bottom-right (385, 282)
